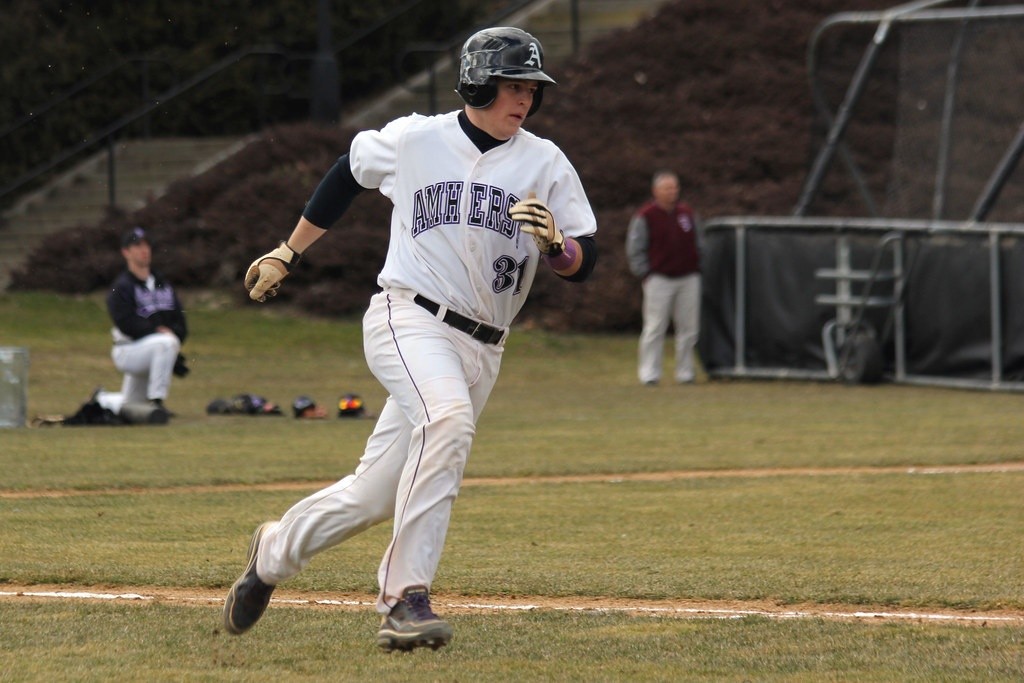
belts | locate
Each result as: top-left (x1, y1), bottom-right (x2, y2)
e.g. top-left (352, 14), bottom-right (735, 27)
top-left (415, 296), bottom-right (503, 345)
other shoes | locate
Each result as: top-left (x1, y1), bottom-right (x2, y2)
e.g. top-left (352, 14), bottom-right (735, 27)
top-left (148, 399), bottom-right (173, 417)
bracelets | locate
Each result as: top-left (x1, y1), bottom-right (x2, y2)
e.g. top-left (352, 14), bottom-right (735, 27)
top-left (543, 238), bottom-right (576, 270)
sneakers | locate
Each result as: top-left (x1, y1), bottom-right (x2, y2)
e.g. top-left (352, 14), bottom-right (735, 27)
top-left (377, 585), bottom-right (453, 653)
top-left (223, 521), bottom-right (278, 635)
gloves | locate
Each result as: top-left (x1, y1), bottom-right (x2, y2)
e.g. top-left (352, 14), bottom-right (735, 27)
top-left (510, 191), bottom-right (566, 256)
top-left (243, 240), bottom-right (303, 303)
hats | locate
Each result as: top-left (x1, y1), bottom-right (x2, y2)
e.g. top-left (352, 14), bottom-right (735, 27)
top-left (121, 227), bottom-right (148, 248)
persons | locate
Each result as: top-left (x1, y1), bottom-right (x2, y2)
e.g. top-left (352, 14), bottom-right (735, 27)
top-left (225, 26), bottom-right (596, 652)
top-left (91, 228), bottom-right (190, 416)
top-left (627, 172), bottom-right (702, 385)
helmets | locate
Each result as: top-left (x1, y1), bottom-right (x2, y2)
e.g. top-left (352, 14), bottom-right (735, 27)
top-left (454, 27), bottom-right (559, 118)
